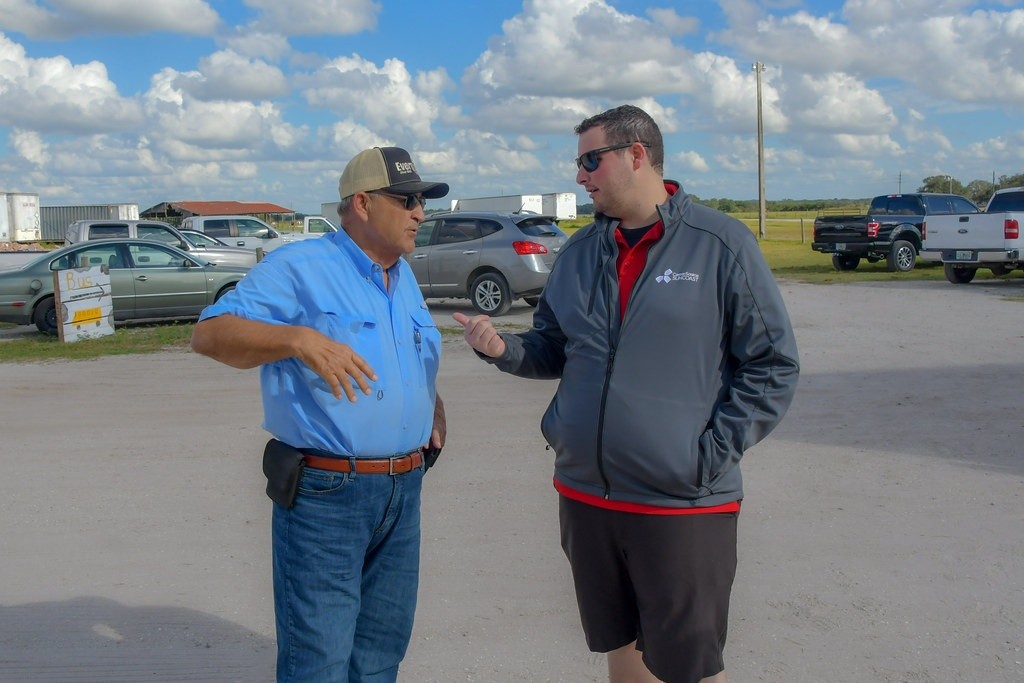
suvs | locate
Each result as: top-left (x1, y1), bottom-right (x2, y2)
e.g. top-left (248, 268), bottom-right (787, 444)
top-left (400, 210), bottom-right (569, 317)
top-left (181, 216), bottom-right (324, 254)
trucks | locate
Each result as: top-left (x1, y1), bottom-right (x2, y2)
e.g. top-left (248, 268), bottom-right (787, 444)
top-left (304, 216), bottom-right (338, 236)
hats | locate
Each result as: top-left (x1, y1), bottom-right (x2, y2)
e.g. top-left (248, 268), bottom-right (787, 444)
top-left (338, 147), bottom-right (450, 199)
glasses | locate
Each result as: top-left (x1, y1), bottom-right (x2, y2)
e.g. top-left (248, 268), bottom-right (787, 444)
top-left (575, 141), bottom-right (652, 172)
top-left (364, 191), bottom-right (427, 212)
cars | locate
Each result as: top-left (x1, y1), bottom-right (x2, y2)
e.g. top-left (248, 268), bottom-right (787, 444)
top-left (0, 238), bottom-right (252, 336)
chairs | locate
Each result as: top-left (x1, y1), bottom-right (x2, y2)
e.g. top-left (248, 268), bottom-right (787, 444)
top-left (108, 254), bottom-right (117, 269)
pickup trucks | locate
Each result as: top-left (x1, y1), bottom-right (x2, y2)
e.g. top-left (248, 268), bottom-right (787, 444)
top-left (921, 187), bottom-right (1024, 284)
top-left (64, 219), bottom-right (268, 269)
top-left (811, 192), bottom-right (982, 272)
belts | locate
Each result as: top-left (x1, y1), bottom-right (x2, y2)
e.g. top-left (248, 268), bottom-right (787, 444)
top-left (303, 450), bottom-right (425, 475)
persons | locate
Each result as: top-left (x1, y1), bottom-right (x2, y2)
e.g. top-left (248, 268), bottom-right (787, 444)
top-left (188, 146), bottom-right (449, 683)
top-left (452, 106), bottom-right (801, 683)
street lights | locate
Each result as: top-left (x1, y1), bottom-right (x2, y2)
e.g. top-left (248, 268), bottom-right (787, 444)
top-left (753, 60), bottom-right (767, 240)
